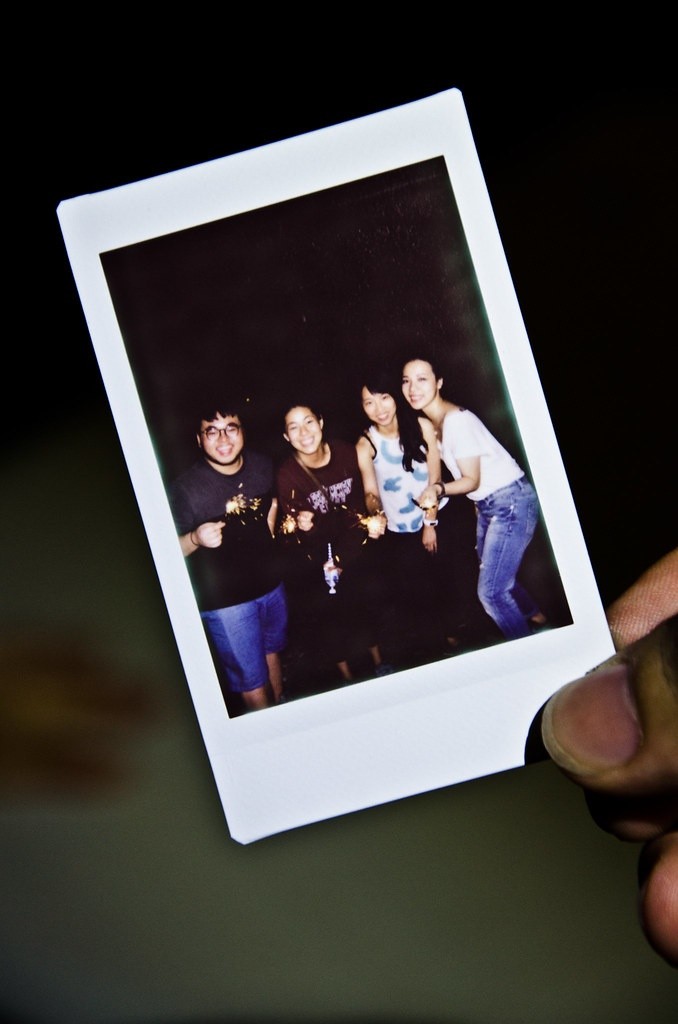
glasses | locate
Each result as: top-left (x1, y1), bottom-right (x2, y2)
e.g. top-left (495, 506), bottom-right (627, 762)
top-left (201, 422), bottom-right (242, 441)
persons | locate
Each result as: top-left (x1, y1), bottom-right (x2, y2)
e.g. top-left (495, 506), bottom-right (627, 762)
top-left (167, 352), bottom-right (548, 713)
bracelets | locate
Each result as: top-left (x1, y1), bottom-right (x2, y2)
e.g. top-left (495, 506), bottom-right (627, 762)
top-left (191, 531), bottom-right (198, 546)
top-left (435, 482), bottom-right (445, 499)
top-left (423, 519), bottom-right (438, 527)
